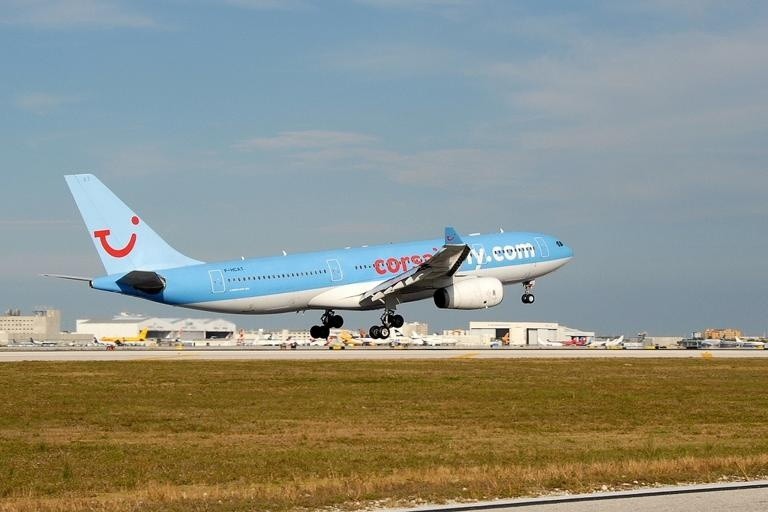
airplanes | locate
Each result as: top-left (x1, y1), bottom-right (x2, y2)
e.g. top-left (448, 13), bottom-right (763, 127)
top-left (1, 326), bottom-right (766, 349)
top-left (40, 172), bottom-right (572, 339)
